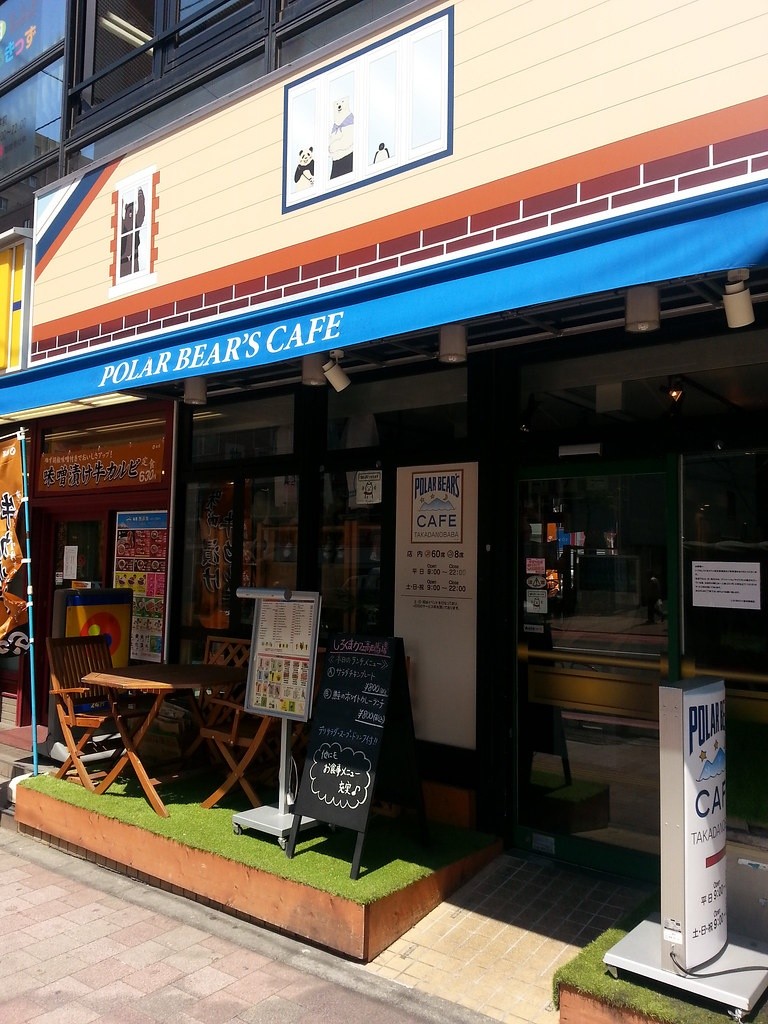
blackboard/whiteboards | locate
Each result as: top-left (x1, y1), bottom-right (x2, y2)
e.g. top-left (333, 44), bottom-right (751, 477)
top-left (290, 634), bottom-right (402, 834)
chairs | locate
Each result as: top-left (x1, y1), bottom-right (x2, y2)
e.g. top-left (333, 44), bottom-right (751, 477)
top-left (48, 635), bottom-right (326, 810)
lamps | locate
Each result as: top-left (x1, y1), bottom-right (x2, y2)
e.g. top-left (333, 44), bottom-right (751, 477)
top-left (322, 350), bottom-right (351, 392)
top-left (721, 268), bottom-right (753, 327)
top-left (625, 286), bottom-right (659, 334)
top-left (182, 376), bottom-right (208, 404)
top-left (438, 324), bottom-right (467, 362)
top-left (302, 354), bottom-right (327, 385)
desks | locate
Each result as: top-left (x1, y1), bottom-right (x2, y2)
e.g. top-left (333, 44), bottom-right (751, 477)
top-left (80, 664), bottom-right (248, 818)
